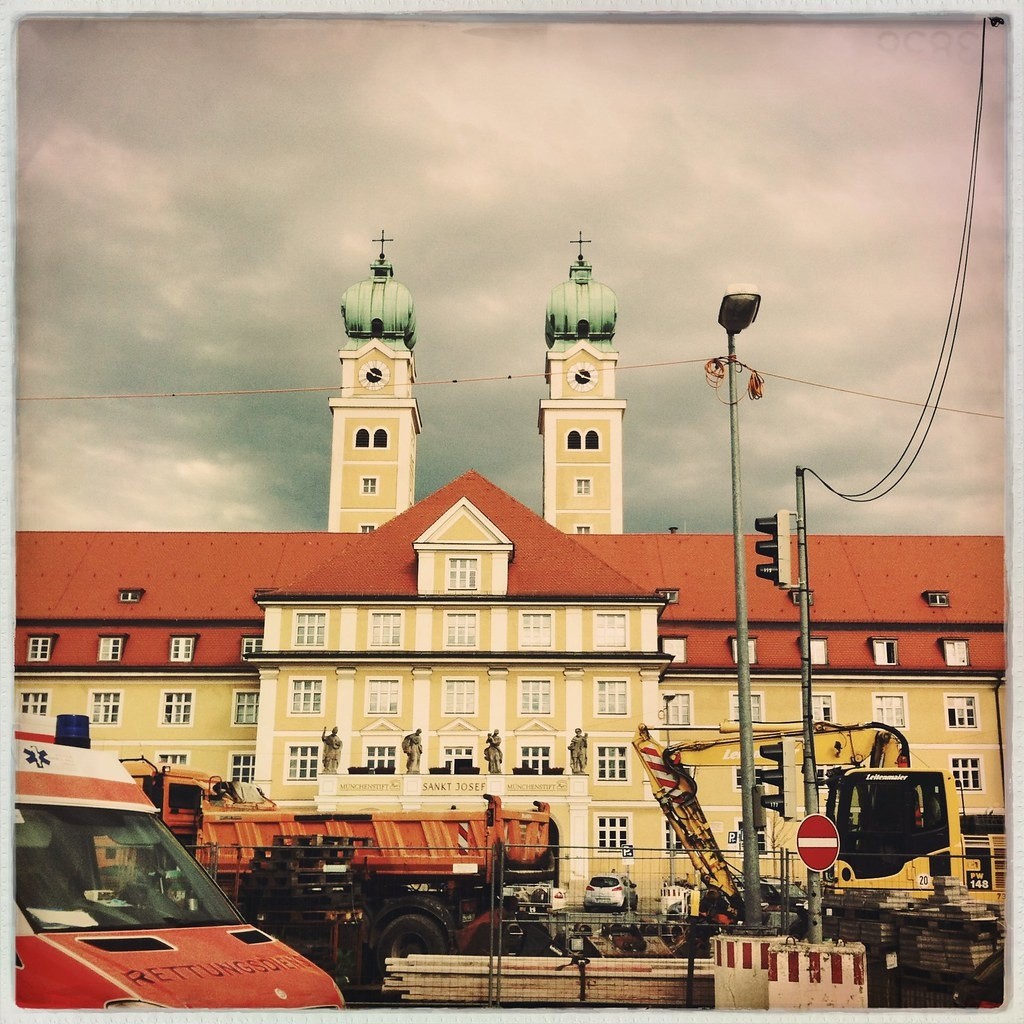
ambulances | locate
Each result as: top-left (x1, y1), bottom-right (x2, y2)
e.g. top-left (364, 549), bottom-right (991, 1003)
top-left (16, 711), bottom-right (349, 1011)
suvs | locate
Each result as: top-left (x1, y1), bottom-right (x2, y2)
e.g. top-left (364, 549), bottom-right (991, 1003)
top-left (582, 873), bottom-right (639, 913)
top-left (731, 875), bottom-right (809, 904)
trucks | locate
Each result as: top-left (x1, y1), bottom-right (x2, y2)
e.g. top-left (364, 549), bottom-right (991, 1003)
top-left (118, 756), bottom-right (556, 985)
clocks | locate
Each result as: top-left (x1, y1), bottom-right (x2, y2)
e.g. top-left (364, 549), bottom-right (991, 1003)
top-left (359, 361), bottom-right (391, 390)
top-left (567, 361), bottom-right (598, 392)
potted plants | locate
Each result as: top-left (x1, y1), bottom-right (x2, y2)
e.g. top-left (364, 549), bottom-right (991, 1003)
top-left (456, 767), bottom-right (480, 775)
top-left (348, 766), bottom-right (370, 774)
top-left (375, 765), bottom-right (395, 774)
top-left (542, 767), bottom-right (564, 776)
top-left (429, 767), bottom-right (450, 774)
top-left (512, 767), bottom-right (533, 775)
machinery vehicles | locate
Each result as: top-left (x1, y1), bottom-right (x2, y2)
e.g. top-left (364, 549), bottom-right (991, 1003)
top-left (632, 720), bottom-right (1005, 925)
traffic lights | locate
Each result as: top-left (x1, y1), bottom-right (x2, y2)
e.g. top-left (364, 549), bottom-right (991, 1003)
top-left (753, 509), bottom-right (792, 588)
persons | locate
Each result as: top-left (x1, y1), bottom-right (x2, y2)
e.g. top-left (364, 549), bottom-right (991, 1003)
top-left (322, 726), bottom-right (343, 771)
top-left (484, 729), bottom-right (503, 773)
top-left (568, 728), bottom-right (588, 773)
top-left (402, 729), bottom-right (423, 772)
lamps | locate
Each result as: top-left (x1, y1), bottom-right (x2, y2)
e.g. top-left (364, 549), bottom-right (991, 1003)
top-left (835, 741), bottom-right (842, 758)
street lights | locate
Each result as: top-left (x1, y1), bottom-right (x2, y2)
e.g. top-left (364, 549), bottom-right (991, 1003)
top-left (716, 281), bottom-right (764, 937)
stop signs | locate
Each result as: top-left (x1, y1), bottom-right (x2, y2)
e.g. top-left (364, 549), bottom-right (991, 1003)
top-left (797, 811), bottom-right (841, 873)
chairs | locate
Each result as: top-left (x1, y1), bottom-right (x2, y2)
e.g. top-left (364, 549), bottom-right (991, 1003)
top-left (19, 822), bottom-right (86, 911)
top-left (852, 788), bottom-right (918, 869)
